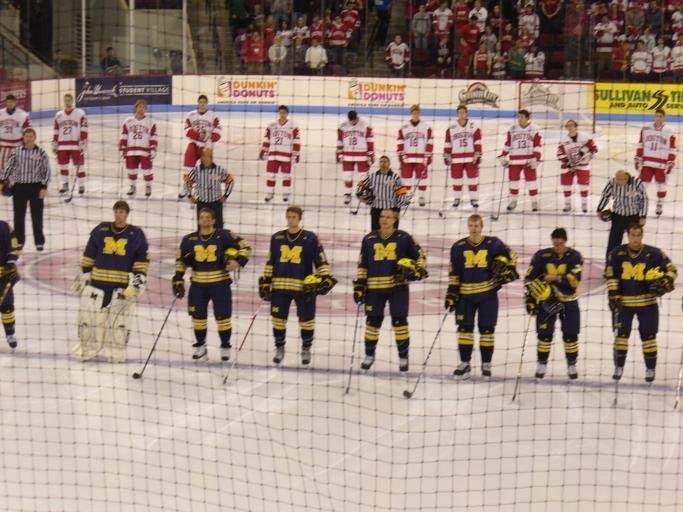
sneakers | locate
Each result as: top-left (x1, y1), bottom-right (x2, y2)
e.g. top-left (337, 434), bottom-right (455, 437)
top-left (564, 204), bottom-right (573, 211)
top-left (470, 200), bottom-right (479, 209)
top-left (60, 184), bottom-right (69, 194)
top-left (582, 203), bottom-right (587, 213)
top-left (264, 193), bottom-right (274, 201)
top-left (176, 189), bottom-right (187, 200)
top-left (343, 193), bottom-right (352, 208)
top-left (282, 193), bottom-right (290, 203)
top-left (145, 186), bottom-right (151, 199)
top-left (507, 200), bottom-right (517, 211)
top-left (126, 186), bottom-right (136, 196)
top-left (531, 201), bottom-right (537, 212)
top-left (419, 197), bottom-right (425, 207)
top-left (452, 198), bottom-right (460, 207)
top-left (78, 185), bottom-right (85, 194)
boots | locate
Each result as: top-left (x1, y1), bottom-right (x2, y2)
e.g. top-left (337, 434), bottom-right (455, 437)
top-left (645, 365), bottom-right (656, 383)
top-left (273, 345), bottom-right (285, 364)
top-left (361, 352), bottom-right (375, 369)
top-left (191, 342), bottom-right (208, 361)
top-left (566, 359), bottom-right (578, 379)
top-left (535, 360), bottom-right (547, 379)
top-left (300, 344), bottom-right (313, 365)
top-left (18, 243), bottom-right (45, 251)
top-left (221, 343), bottom-right (232, 361)
top-left (481, 361), bottom-right (492, 377)
top-left (6, 334), bottom-right (17, 349)
top-left (399, 353), bottom-right (409, 372)
top-left (655, 204), bottom-right (663, 216)
top-left (611, 364), bottom-right (625, 381)
top-left (453, 360), bottom-right (471, 376)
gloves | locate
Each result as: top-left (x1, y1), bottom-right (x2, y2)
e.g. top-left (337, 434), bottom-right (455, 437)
top-left (524, 295), bottom-right (537, 315)
top-left (442, 284), bottom-right (460, 313)
top-left (663, 160), bottom-right (674, 174)
top-left (635, 154), bottom-right (642, 171)
top-left (353, 277), bottom-right (368, 305)
top-left (319, 276), bottom-right (338, 296)
top-left (255, 275), bottom-right (273, 301)
top-left (172, 273), bottom-right (186, 299)
top-left (409, 267), bottom-right (428, 281)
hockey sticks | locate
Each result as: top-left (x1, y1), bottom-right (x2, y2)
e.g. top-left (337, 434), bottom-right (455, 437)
top-left (403, 305), bottom-right (451, 398)
top-left (437, 167), bottom-right (450, 218)
top-left (222, 296), bottom-right (265, 386)
top-left (131, 291), bottom-right (683, 411)
top-left (511, 303), bottom-right (535, 402)
top-left (64, 151), bottom-right (84, 204)
top-left (612, 299), bottom-right (620, 407)
top-left (342, 301), bottom-right (363, 399)
top-left (351, 165), bottom-right (373, 215)
top-left (491, 167), bottom-right (507, 221)
top-left (673, 348), bottom-right (683, 409)
top-left (132, 291), bottom-right (179, 379)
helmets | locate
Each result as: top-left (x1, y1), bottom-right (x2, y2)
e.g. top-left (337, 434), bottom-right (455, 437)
top-left (221, 246), bottom-right (238, 266)
top-left (395, 257), bottom-right (419, 279)
top-left (643, 265), bottom-right (667, 283)
top-left (601, 208), bottom-right (614, 222)
top-left (303, 273), bottom-right (321, 294)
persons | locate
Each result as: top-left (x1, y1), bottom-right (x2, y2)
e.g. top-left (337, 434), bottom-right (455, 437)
top-left (353, 209), bottom-right (429, 377)
top-left (596, 169), bottom-right (647, 276)
top-left (171, 209), bottom-right (252, 362)
top-left (443, 105), bottom-right (482, 208)
top-left (0, 220), bottom-right (19, 349)
top-left (335, 111), bottom-right (374, 205)
top-left (183, 149), bottom-right (234, 228)
top-left (524, 228), bottom-right (585, 381)
top-left (355, 156), bottom-right (407, 232)
top-left (177, 95), bottom-right (224, 201)
top-left (259, 206), bottom-right (337, 364)
top-left (69, 201), bottom-right (149, 364)
top-left (3, 128), bottom-right (52, 251)
top-left (258, 105), bottom-right (301, 201)
top-left (603, 223), bottom-right (677, 382)
top-left (635, 109), bottom-right (677, 217)
top-left (497, 109), bottom-right (543, 210)
top-left (443, 215), bottom-right (521, 380)
top-left (118, 100), bottom-right (158, 198)
top-left (556, 120), bottom-right (598, 212)
top-left (1, 94), bottom-right (32, 185)
top-left (238, 1), bottom-right (683, 82)
top-left (396, 105), bottom-right (434, 206)
top-left (50, 94), bottom-right (88, 196)
top-left (52, 50), bottom-right (76, 77)
top-left (102, 47), bottom-right (123, 75)
